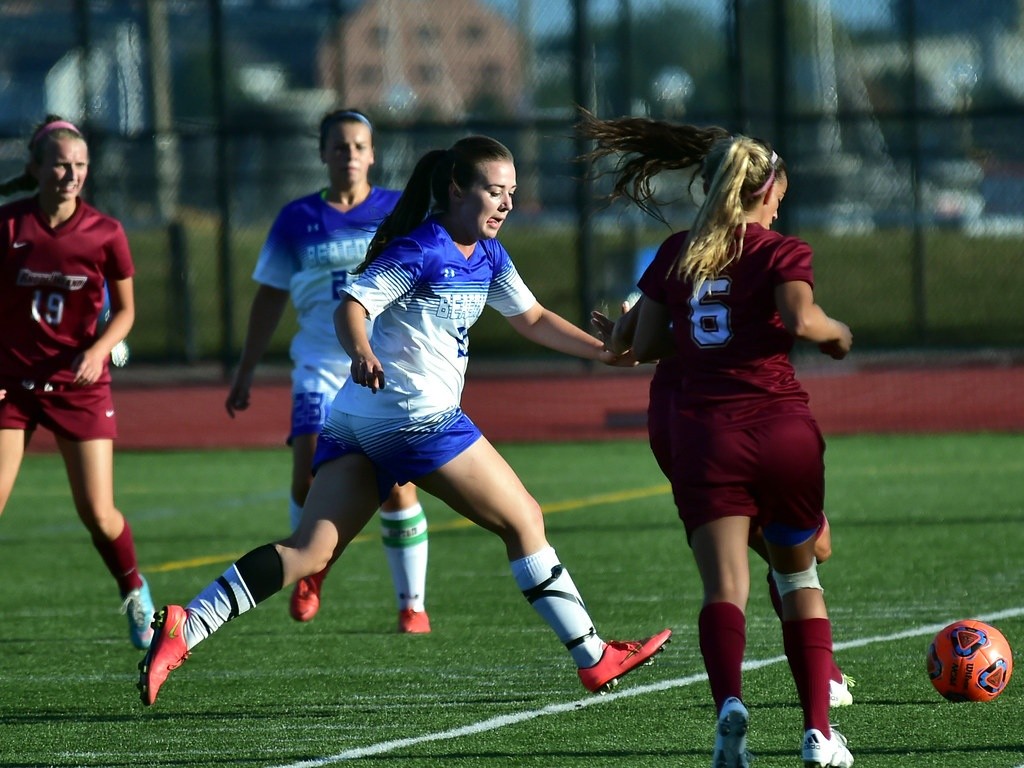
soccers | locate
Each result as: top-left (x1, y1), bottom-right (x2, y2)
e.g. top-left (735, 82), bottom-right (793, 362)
top-left (927, 620), bottom-right (1014, 703)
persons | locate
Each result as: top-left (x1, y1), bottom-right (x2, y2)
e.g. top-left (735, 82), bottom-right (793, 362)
top-left (0, 112), bottom-right (157, 649)
top-left (137, 135), bottom-right (671, 707)
top-left (570, 114), bottom-right (855, 768)
top-left (225, 111), bottom-right (429, 631)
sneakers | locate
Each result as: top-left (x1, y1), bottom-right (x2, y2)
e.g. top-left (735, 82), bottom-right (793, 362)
top-left (399, 609), bottom-right (430, 633)
top-left (802, 724), bottom-right (854, 768)
top-left (136, 605), bottom-right (192, 706)
top-left (829, 674), bottom-right (856, 708)
top-left (119, 574), bottom-right (156, 649)
top-left (290, 569), bottom-right (326, 621)
top-left (712, 697), bottom-right (750, 768)
top-left (578, 629), bottom-right (672, 695)
